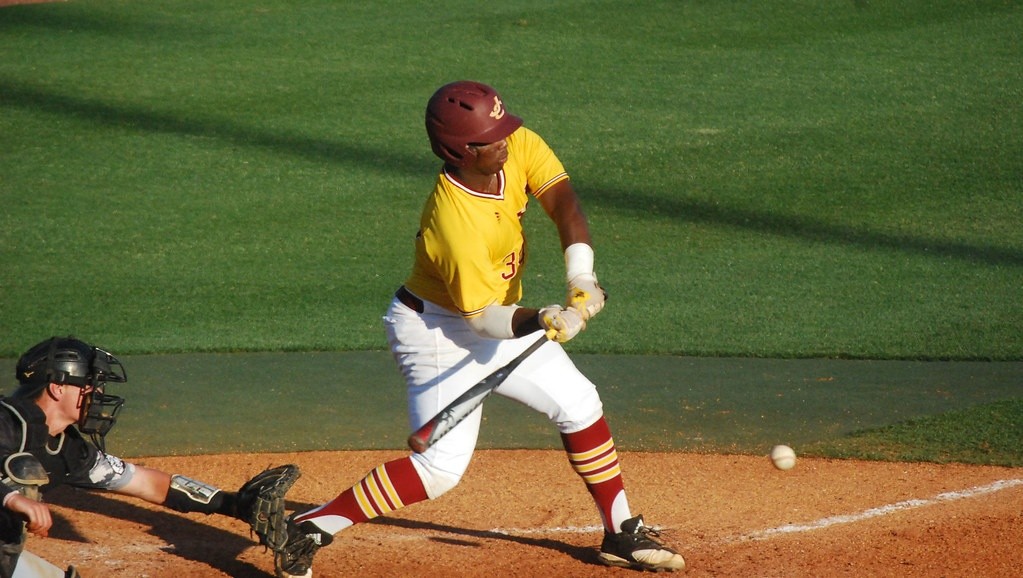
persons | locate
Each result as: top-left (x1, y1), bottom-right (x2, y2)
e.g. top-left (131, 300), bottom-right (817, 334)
top-left (0, 335), bottom-right (302, 578)
top-left (277, 80), bottom-right (688, 578)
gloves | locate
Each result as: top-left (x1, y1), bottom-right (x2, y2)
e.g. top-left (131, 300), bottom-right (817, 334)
top-left (567, 272), bottom-right (605, 330)
top-left (538, 303), bottom-right (583, 343)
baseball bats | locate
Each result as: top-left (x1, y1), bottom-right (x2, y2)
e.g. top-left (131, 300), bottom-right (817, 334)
top-left (407, 285), bottom-right (610, 455)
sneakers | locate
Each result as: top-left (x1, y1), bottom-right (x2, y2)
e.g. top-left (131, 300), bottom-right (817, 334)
top-left (274, 503), bottom-right (334, 578)
top-left (597, 514), bottom-right (685, 572)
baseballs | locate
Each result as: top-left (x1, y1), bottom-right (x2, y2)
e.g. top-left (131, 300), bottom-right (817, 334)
top-left (771, 444), bottom-right (797, 471)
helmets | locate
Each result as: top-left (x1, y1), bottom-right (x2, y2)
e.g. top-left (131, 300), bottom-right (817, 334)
top-left (15, 336), bottom-right (112, 389)
top-left (424, 81), bottom-right (524, 166)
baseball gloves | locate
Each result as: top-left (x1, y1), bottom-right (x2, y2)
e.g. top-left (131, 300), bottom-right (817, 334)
top-left (239, 460), bottom-right (301, 558)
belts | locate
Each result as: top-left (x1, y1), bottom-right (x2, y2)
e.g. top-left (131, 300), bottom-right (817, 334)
top-left (394, 285), bottom-right (425, 314)
